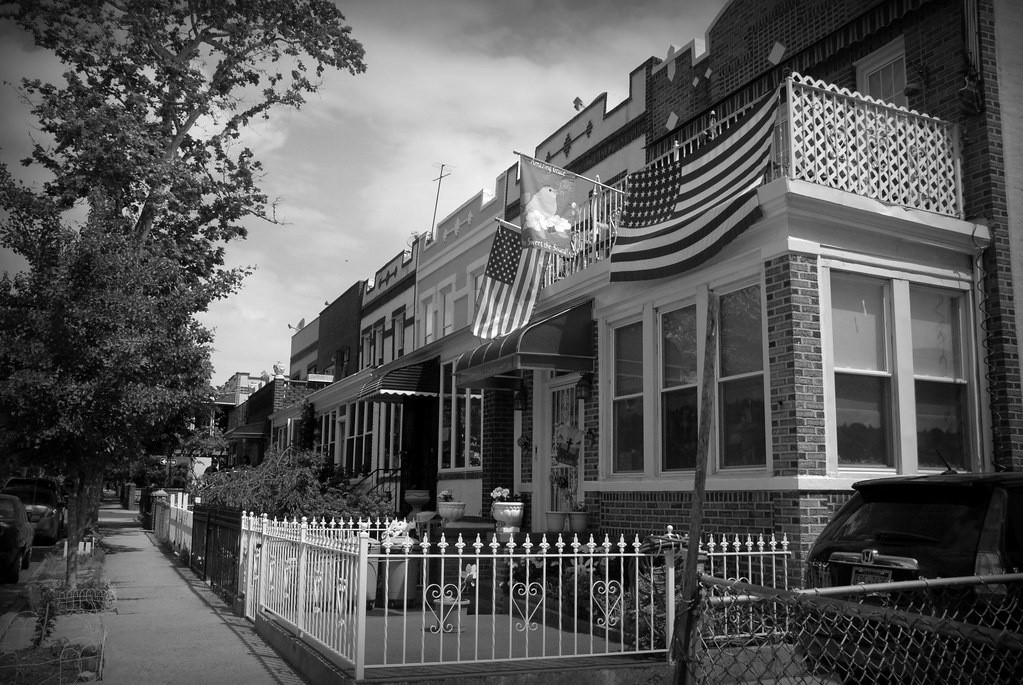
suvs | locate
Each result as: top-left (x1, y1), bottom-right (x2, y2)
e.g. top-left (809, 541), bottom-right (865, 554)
top-left (793, 472), bottom-right (1023, 685)
top-left (0, 478), bottom-right (65, 544)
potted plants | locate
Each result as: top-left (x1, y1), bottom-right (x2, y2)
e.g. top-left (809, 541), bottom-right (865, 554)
top-left (566, 489), bottom-right (589, 532)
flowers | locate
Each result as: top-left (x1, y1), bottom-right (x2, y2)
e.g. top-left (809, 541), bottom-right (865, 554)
top-left (437, 488), bottom-right (455, 502)
top-left (490, 487), bottom-right (510, 531)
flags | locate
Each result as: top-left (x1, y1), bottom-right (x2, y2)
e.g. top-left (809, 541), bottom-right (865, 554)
top-left (469, 224), bottom-right (550, 340)
top-left (519, 154), bottom-right (577, 259)
top-left (607, 82), bottom-right (780, 283)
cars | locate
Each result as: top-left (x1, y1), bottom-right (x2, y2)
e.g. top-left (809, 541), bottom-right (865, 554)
top-left (0, 494), bottom-right (34, 582)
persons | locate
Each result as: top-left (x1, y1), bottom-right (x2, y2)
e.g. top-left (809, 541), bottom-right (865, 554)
top-left (204, 459), bottom-right (218, 473)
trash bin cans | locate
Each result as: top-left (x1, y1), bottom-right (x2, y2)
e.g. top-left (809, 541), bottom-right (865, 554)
top-left (341, 537), bottom-right (382, 602)
top-left (382, 536), bottom-right (422, 602)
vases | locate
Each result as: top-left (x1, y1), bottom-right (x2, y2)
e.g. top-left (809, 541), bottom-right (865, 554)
top-left (434, 598), bottom-right (471, 632)
top-left (545, 511), bottom-right (567, 532)
top-left (493, 501), bottom-right (524, 532)
top-left (438, 502), bottom-right (466, 522)
